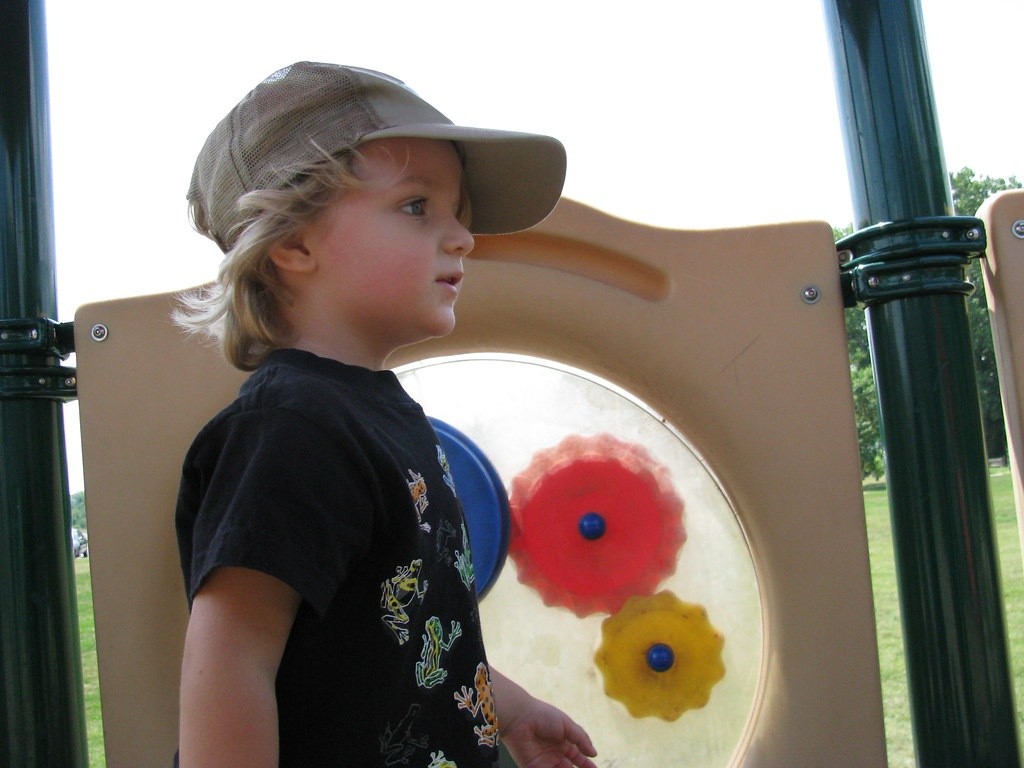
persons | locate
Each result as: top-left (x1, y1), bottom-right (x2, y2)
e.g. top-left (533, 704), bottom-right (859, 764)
top-left (171, 61), bottom-right (597, 768)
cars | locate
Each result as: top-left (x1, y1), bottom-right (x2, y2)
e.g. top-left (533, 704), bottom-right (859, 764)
top-left (72, 529), bottom-right (87, 558)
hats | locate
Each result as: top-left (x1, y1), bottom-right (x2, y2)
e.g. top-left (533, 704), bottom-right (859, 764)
top-left (188, 62), bottom-right (566, 250)
